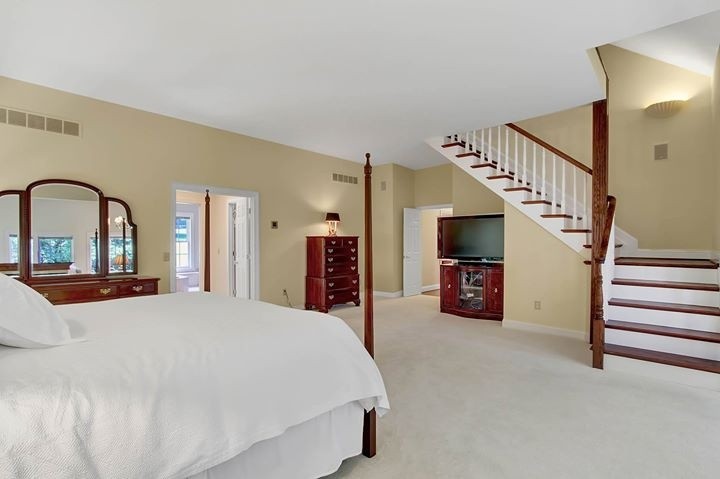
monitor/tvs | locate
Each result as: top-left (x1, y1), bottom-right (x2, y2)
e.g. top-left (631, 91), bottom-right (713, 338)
top-left (436, 213), bottom-right (504, 264)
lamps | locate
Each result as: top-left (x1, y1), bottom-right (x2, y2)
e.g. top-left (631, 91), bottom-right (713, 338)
top-left (644, 100), bottom-right (687, 118)
top-left (325, 213), bottom-right (340, 236)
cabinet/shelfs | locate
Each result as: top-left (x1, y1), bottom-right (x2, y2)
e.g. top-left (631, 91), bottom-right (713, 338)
top-left (306, 236), bottom-right (361, 313)
top-left (439, 264), bottom-right (503, 321)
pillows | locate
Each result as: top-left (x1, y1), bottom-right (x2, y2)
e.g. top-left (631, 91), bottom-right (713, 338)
top-left (0, 272), bottom-right (73, 348)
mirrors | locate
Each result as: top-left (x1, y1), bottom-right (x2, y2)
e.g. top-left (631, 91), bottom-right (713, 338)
top-left (108, 201), bottom-right (135, 273)
top-left (0, 194), bottom-right (21, 279)
top-left (29, 183), bottom-right (102, 277)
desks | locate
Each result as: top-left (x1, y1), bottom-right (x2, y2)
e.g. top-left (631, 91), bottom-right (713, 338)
top-left (30, 276), bottom-right (160, 304)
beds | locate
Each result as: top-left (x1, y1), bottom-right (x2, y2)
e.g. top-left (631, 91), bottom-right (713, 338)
top-left (0, 291), bottom-right (391, 479)
